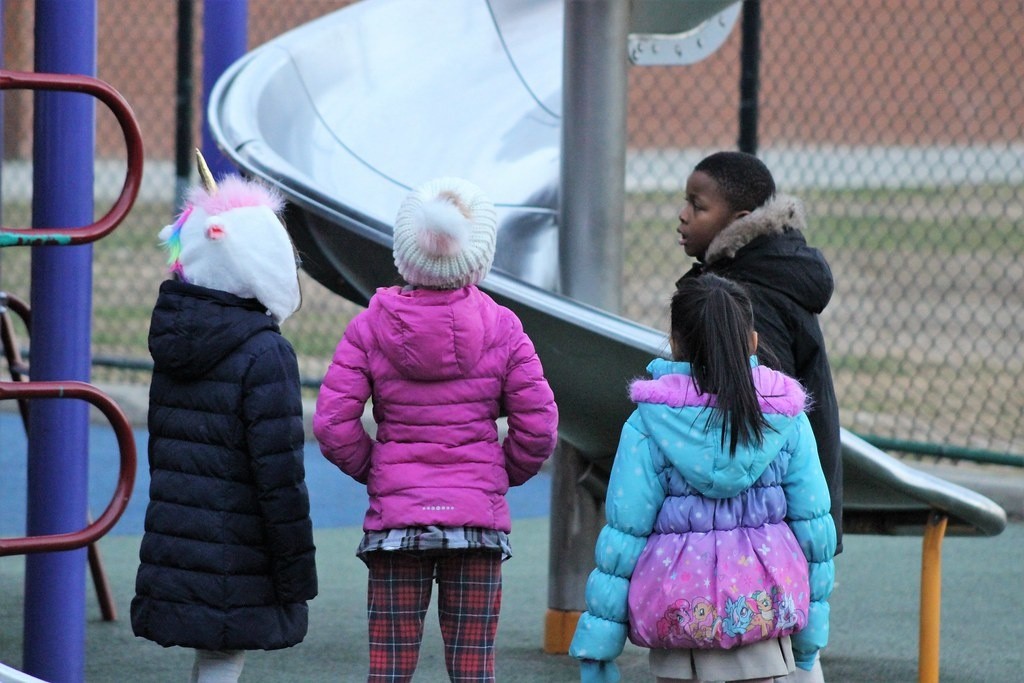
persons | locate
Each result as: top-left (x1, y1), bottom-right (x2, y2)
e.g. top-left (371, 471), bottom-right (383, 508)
top-left (130, 174), bottom-right (319, 683)
top-left (568, 273), bottom-right (837, 683)
top-left (314, 178), bottom-right (559, 683)
top-left (678, 151), bottom-right (844, 683)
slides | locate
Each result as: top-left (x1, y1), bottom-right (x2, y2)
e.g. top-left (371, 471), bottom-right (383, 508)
top-left (204, 0), bottom-right (1011, 540)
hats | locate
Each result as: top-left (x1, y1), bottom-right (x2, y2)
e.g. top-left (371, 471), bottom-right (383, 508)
top-left (392, 176), bottom-right (500, 289)
top-left (156, 171), bottom-right (301, 327)
